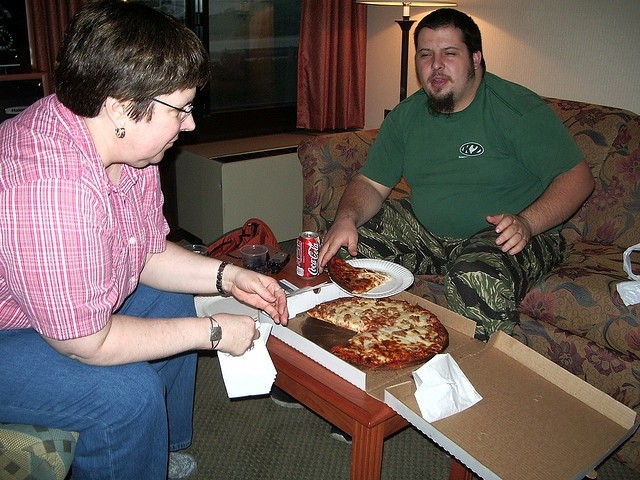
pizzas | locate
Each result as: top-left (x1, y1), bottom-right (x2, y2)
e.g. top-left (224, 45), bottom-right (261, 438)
top-left (307, 296), bottom-right (450, 371)
top-left (327, 256), bottom-right (392, 295)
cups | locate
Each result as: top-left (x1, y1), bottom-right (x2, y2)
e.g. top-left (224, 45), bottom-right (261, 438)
top-left (184, 244), bottom-right (209, 257)
top-left (239, 244), bottom-right (269, 275)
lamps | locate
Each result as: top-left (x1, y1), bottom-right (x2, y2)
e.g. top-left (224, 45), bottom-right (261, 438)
top-left (361, 0), bottom-right (459, 119)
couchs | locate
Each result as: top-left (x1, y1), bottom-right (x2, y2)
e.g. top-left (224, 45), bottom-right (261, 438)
top-left (0, 420), bottom-right (79, 480)
top-left (296, 93), bottom-right (640, 476)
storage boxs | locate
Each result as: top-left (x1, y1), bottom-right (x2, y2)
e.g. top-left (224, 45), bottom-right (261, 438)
top-left (257, 283), bottom-right (640, 479)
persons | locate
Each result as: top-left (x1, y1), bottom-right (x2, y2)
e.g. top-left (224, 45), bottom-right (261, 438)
top-left (318, 9), bottom-right (596, 344)
top-left (2, 1), bottom-right (288, 479)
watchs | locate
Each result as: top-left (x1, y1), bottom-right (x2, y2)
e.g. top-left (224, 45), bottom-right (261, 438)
top-left (216, 261), bottom-right (234, 297)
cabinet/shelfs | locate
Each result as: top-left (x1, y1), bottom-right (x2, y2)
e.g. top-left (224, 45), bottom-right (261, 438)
top-left (0, 67), bottom-right (51, 128)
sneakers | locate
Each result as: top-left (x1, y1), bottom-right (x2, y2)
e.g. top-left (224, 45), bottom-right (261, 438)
top-left (332, 427), bottom-right (353, 444)
top-left (168, 452), bottom-right (196, 479)
top-left (271, 389), bottom-right (304, 409)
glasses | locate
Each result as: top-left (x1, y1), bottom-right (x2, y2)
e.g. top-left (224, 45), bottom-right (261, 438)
top-left (154, 95), bottom-right (193, 121)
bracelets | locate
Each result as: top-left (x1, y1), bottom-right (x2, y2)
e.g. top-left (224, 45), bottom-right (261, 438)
top-left (204, 316), bottom-right (222, 350)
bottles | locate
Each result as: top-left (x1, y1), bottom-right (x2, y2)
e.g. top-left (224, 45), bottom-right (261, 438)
top-left (295, 231), bottom-right (321, 281)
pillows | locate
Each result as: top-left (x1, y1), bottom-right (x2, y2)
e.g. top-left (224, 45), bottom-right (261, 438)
top-left (522, 237), bottom-right (640, 362)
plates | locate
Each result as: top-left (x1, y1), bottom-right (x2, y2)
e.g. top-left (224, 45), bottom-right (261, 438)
top-left (329, 259), bottom-right (416, 298)
